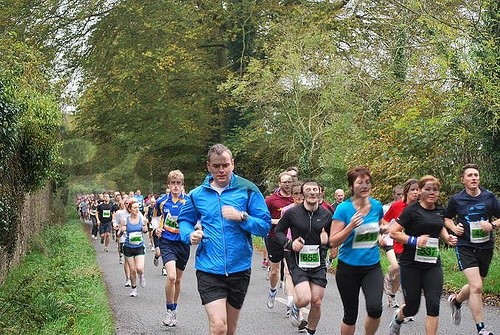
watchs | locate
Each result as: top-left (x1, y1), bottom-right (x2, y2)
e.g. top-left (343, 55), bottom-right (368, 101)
top-left (240, 211), bottom-right (248, 223)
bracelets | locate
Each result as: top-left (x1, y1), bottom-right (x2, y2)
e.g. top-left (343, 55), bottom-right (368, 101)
top-left (407, 236), bottom-right (416, 247)
top-left (491, 221), bottom-right (496, 229)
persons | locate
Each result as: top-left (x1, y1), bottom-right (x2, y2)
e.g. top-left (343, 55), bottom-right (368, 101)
top-left (383, 176), bottom-right (458, 335)
top-left (444, 164), bottom-right (500, 335)
top-left (265, 167), bottom-right (345, 335)
top-left (153, 170), bottom-right (190, 327)
top-left (76, 190), bottom-right (168, 297)
top-left (329, 167), bottom-right (386, 335)
top-left (177, 144), bottom-right (272, 335)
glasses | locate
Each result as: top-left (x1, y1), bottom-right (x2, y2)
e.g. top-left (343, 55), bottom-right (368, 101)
top-left (281, 181), bottom-right (292, 184)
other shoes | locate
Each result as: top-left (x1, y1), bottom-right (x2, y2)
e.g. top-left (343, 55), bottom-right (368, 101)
top-left (279, 281), bottom-right (283, 288)
top-left (261, 258), bottom-right (267, 269)
top-left (401, 304), bottom-right (414, 322)
top-left (125, 278), bottom-right (132, 287)
top-left (151, 246), bottom-right (155, 252)
top-left (130, 286), bottom-right (138, 297)
top-left (139, 276), bottom-right (146, 288)
top-left (91, 235), bottom-right (97, 240)
top-left (101, 238), bottom-right (104, 244)
top-left (286, 306), bottom-right (290, 317)
top-left (104, 247), bottom-right (109, 252)
top-left (119, 256), bottom-right (123, 264)
top-left (298, 316), bottom-right (308, 330)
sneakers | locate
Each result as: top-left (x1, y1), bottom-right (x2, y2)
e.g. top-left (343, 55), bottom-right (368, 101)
top-left (266, 288), bottom-right (278, 309)
top-left (154, 256), bottom-right (160, 266)
top-left (289, 301), bottom-right (301, 327)
top-left (162, 267), bottom-right (167, 276)
top-left (448, 293), bottom-right (463, 325)
top-left (383, 276), bottom-right (392, 295)
top-left (388, 308), bottom-right (405, 335)
top-left (477, 328), bottom-right (495, 335)
top-left (326, 253), bottom-right (334, 270)
top-left (162, 309), bottom-right (179, 328)
top-left (387, 295), bottom-right (399, 308)
